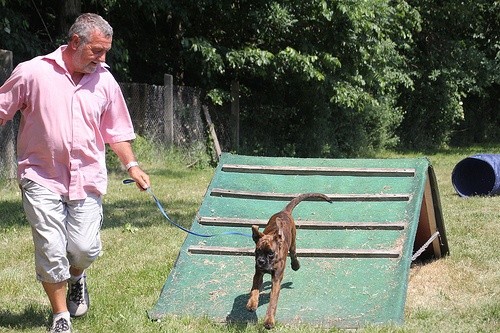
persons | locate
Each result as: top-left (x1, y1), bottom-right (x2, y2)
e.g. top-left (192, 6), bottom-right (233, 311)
top-left (0, 13), bottom-right (150, 333)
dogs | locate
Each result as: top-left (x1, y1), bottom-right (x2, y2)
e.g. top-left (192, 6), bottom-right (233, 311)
top-left (246, 193), bottom-right (332, 329)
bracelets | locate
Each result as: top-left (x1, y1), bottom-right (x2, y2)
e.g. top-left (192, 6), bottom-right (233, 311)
top-left (125, 161), bottom-right (139, 172)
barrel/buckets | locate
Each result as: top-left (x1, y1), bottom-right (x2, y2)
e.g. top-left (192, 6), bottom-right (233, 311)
top-left (452, 154), bottom-right (500, 198)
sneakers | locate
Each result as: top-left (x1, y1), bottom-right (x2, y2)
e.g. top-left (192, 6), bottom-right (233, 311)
top-left (66, 279), bottom-right (89, 317)
top-left (50, 316), bottom-right (71, 333)
